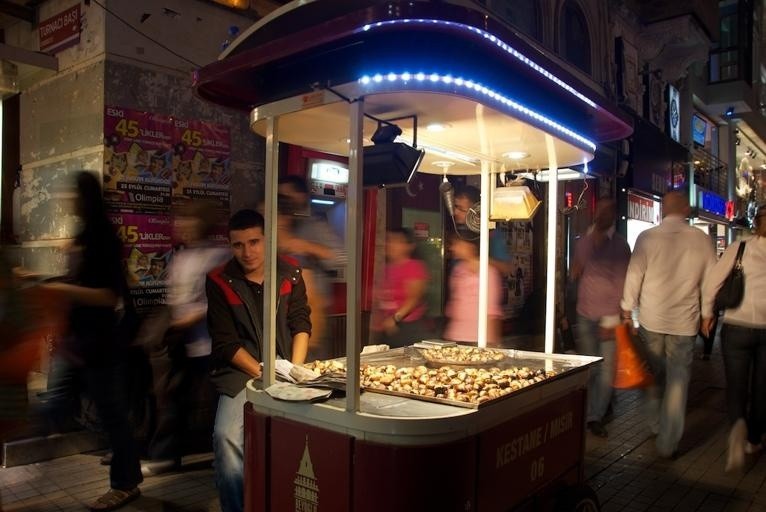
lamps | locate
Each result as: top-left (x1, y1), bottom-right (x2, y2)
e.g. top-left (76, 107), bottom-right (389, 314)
top-left (490, 169), bottom-right (543, 223)
top-left (359, 123), bottom-right (425, 185)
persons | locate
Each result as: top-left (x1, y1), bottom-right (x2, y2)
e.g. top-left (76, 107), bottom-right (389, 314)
top-left (14, 171), bottom-right (347, 511)
top-left (571, 191), bottom-right (766, 470)
top-left (368, 187), bottom-right (509, 345)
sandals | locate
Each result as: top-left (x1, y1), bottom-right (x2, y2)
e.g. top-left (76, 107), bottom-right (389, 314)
top-left (87, 486), bottom-right (141, 512)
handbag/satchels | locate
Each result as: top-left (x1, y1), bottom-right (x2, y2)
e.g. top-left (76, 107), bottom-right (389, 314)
top-left (613, 316), bottom-right (656, 390)
top-left (713, 238), bottom-right (747, 317)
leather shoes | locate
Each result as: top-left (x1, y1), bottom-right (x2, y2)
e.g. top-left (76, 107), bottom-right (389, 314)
top-left (587, 420), bottom-right (609, 438)
